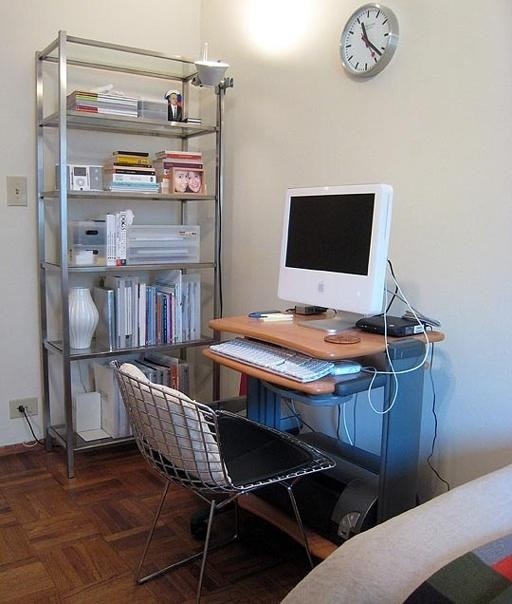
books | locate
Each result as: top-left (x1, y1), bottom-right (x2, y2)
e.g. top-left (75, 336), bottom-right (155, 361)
top-left (87, 211), bottom-right (126, 267)
top-left (183, 118), bottom-right (202, 126)
top-left (152, 150), bottom-right (203, 193)
top-left (102, 150), bottom-right (161, 193)
top-left (92, 269), bottom-right (201, 352)
top-left (94, 354), bottom-right (189, 439)
top-left (67, 90), bottom-right (138, 117)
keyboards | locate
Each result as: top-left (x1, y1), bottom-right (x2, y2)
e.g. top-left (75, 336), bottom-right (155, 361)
top-left (208, 336), bottom-right (335, 384)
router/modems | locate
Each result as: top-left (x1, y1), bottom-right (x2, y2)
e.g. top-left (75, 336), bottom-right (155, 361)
top-left (295, 304), bottom-right (328, 315)
top-left (356, 315), bottom-right (427, 338)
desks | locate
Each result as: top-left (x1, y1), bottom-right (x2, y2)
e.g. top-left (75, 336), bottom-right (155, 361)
top-left (201, 313), bottom-right (445, 561)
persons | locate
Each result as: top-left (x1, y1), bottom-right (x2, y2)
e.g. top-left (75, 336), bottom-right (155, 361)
top-left (168, 93), bottom-right (182, 122)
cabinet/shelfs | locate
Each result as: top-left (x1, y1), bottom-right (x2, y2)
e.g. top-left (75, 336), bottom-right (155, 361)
top-left (30, 28), bottom-right (225, 479)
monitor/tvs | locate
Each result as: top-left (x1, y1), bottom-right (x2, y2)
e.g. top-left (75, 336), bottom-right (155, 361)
top-left (277, 183), bottom-right (394, 333)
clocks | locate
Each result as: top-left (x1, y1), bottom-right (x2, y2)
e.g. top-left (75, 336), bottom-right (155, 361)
top-left (337, 2), bottom-right (399, 80)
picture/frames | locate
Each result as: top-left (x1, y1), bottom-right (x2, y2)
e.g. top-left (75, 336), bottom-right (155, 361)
top-left (169, 166), bottom-right (209, 196)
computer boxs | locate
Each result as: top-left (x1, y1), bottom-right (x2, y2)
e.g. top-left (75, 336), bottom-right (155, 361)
top-left (252, 432), bottom-right (421, 548)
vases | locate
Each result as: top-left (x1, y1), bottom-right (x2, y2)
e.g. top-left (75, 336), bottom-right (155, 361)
top-left (58, 286), bottom-right (101, 350)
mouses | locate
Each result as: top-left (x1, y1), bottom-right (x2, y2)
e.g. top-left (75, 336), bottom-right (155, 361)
top-left (333, 362), bottom-right (361, 376)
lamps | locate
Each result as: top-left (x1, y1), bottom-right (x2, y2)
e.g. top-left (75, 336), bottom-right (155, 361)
top-left (194, 39), bottom-right (230, 89)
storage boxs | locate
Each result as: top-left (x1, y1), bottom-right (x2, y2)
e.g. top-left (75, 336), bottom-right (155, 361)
top-left (67, 218), bottom-right (201, 264)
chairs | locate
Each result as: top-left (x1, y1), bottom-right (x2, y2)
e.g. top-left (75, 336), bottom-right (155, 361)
top-left (108, 358), bottom-right (337, 603)
